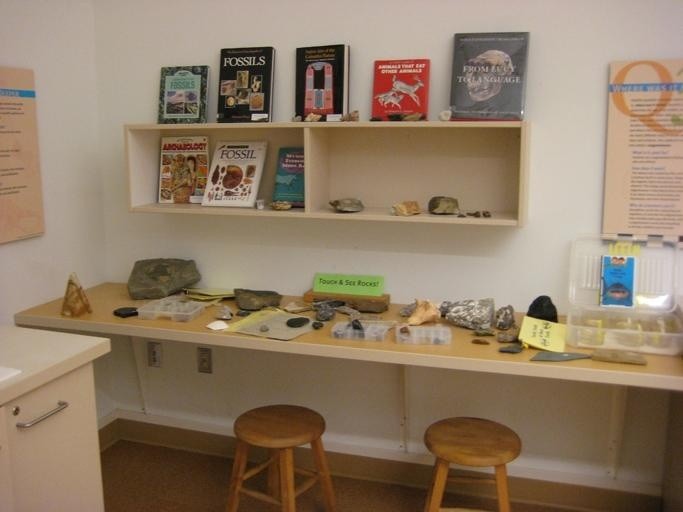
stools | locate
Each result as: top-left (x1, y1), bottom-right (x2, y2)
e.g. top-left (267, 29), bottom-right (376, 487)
top-left (226, 405), bottom-right (335, 512)
top-left (422, 417), bottom-right (521, 511)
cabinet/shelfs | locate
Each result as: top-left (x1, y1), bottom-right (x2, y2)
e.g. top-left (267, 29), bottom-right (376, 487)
top-left (123, 119), bottom-right (530, 227)
top-left (0, 323), bottom-right (112, 511)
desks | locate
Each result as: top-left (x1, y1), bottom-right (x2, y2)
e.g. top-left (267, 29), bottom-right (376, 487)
top-left (15, 282), bottom-right (683, 511)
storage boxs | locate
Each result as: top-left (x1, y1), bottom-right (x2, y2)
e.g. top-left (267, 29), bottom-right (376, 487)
top-left (565, 236), bottom-right (683, 356)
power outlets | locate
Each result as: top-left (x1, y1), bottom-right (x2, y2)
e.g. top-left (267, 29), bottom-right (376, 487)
top-left (196, 346), bottom-right (211, 374)
top-left (147, 343), bottom-right (162, 368)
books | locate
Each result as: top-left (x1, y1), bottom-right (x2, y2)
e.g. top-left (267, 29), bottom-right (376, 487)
top-left (200, 140), bottom-right (268, 208)
top-left (294, 43), bottom-right (350, 121)
top-left (449, 31), bottom-right (530, 121)
top-left (216, 46), bottom-right (275, 123)
top-left (368, 58), bottom-right (431, 122)
top-left (158, 136), bottom-right (210, 204)
top-left (271, 146), bottom-right (304, 208)
top-left (157, 65), bottom-right (209, 123)
